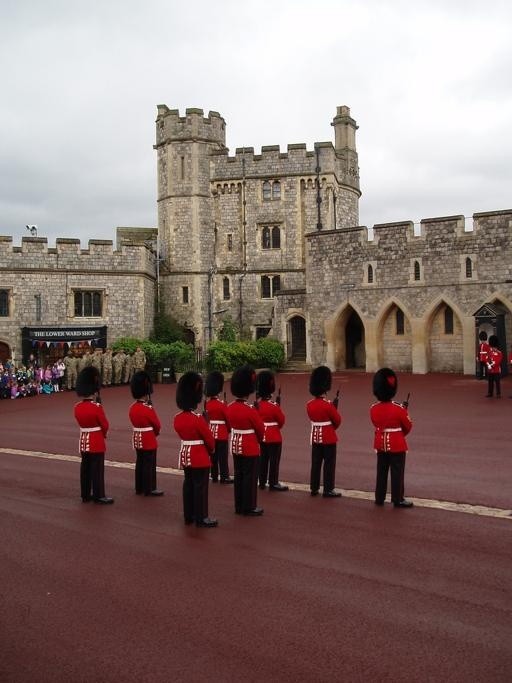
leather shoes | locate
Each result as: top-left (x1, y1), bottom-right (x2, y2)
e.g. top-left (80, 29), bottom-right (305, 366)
top-left (395, 500), bottom-right (413, 508)
top-left (93, 497), bottom-right (114, 504)
top-left (212, 477), bottom-right (218, 482)
top-left (221, 478), bottom-right (234, 482)
top-left (259, 483), bottom-right (265, 489)
top-left (243, 508), bottom-right (263, 517)
top-left (269, 484), bottom-right (288, 491)
top-left (146, 489), bottom-right (164, 496)
top-left (196, 517), bottom-right (217, 527)
top-left (311, 490), bottom-right (319, 495)
top-left (323, 490), bottom-right (341, 497)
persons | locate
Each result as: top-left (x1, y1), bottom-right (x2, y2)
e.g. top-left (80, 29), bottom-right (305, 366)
top-left (305, 365), bottom-right (343, 498)
top-left (222, 365), bottom-right (264, 517)
top-left (201, 371), bottom-right (234, 484)
top-left (0, 344), bottom-right (147, 400)
top-left (506, 344), bottom-right (512, 399)
top-left (473, 329), bottom-right (490, 380)
top-left (482, 334), bottom-right (503, 400)
top-left (171, 372), bottom-right (219, 528)
top-left (368, 366), bottom-right (413, 508)
top-left (74, 366), bottom-right (116, 505)
top-left (129, 370), bottom-right (164, 496)
top-left (253, 370), bottom-right (288, 490)
